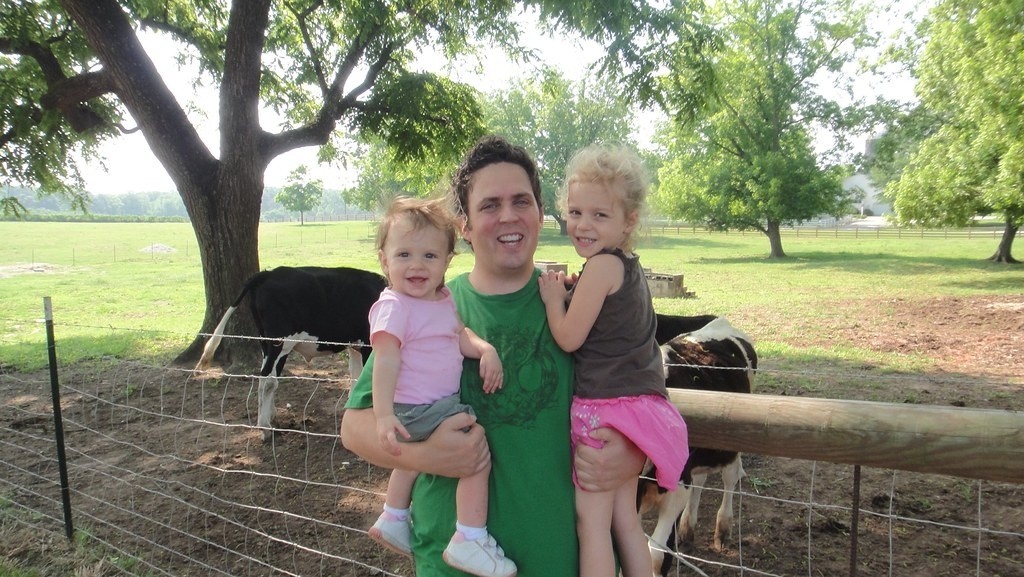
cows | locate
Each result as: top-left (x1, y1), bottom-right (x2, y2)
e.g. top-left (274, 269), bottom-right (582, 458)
top-left (655, 314), bottom-right (722, 347)
top-left (636, 316), bottom-right (760, 577)
top-left (192, 265), bottom-right (391, 444)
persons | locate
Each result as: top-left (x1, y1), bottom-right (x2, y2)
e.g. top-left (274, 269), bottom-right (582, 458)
top-left (340, 139), bottom-right (656, 577)
top-left (539, 140), bottom-right (671, 577)
top-left (367, 197), bottom-right (518, 576)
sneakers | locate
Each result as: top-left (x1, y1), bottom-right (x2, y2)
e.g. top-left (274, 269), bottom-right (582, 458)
top-left (368, 512), bottom-right (413, 557)
top-left (443, 531), bottom-right (517, 577)
